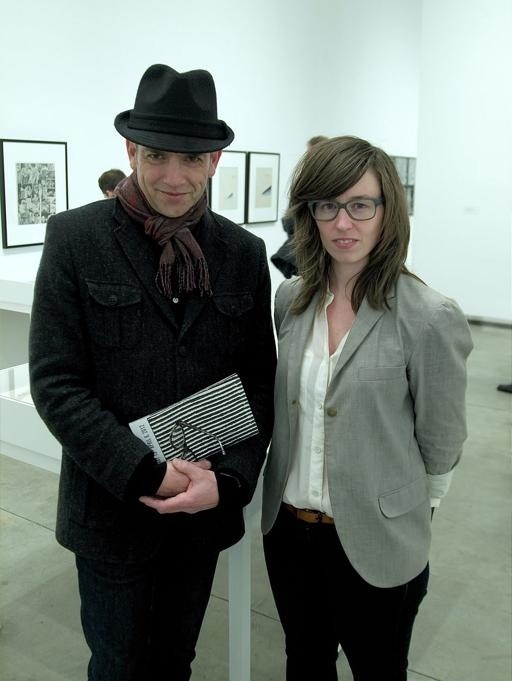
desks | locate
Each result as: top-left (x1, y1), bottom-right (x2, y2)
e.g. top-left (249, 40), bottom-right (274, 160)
top-left (0, 362), bottom-right (272, 681)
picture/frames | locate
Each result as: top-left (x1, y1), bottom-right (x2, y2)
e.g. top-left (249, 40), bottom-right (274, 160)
top-left (0, 138), bottom-right (68, 248)
top-left (243, 152), bottom-right (280, 224)
top-left (209, 150), bottom-right (248, 225)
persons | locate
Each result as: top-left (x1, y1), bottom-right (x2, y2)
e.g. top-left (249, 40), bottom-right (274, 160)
top-left (98, 168), bottom-right (127, 199)
top-left (269, 135), bottom-right (328, 280)
top-left (27, 63), bottom-right (276, 681)
top-left (261, 136), bottom-right (472, 680)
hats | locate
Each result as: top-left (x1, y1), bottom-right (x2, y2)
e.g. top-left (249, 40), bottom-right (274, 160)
top-left (114, 63), bottom-right (237, 154)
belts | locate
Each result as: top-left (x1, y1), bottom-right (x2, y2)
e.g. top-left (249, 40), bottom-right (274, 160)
top-left (279, 500), bottom-right (335, 527)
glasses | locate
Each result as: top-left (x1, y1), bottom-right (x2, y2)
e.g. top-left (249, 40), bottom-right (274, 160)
top-left (166, 421), bottom-right (230, 466)
top-left (311, 195), bottom-right (387, 223)
